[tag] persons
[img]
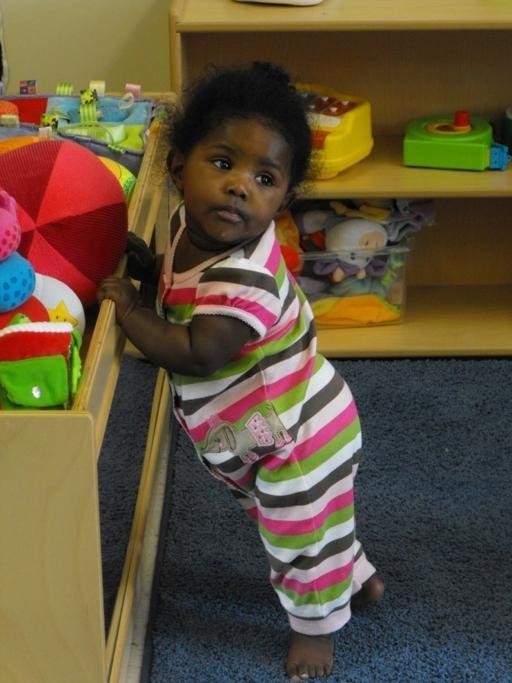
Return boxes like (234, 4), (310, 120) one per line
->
(93, 59), (387, 683)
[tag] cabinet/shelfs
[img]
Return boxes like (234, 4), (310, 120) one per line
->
(171, 1), (510, 356)
(1, 88), (175, 683)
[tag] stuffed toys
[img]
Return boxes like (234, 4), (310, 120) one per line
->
(295, 198), (386, 282)
